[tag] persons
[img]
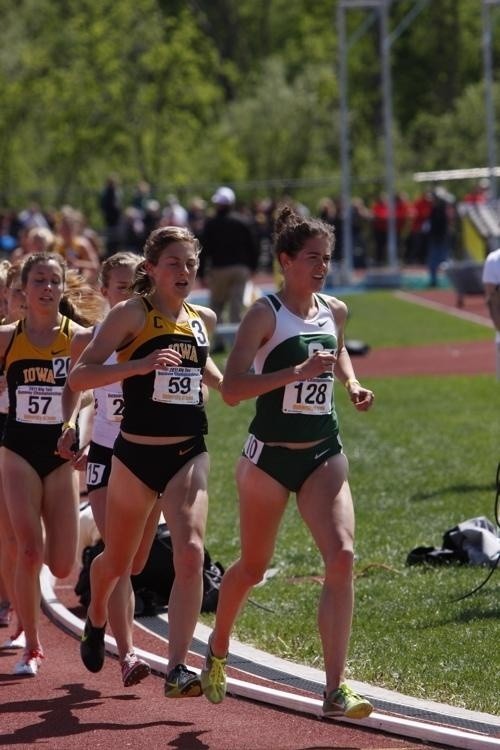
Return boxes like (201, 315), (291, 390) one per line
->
(68, 226), (223, 699)
(0, 258), (39, 649)
(57, 249), (152, 686)
(0, 254), (87, 679)
(200, 204), (374, 723)
(0, 578), (13, 628)
(482, 245), (499, 376)
(2, 173), (499, 327)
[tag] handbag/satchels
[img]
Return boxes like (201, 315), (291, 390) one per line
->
(405, 516), (500, 568)
(75, 522), (224, 618)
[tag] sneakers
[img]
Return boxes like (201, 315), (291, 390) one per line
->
(0, 623), (26, 648)
(80, 617), (107, 673)
(164, 667), (203, 698)
(121, 653), (151, 687)
(200, 633), (230, 704)
(13, 646), (43, 675)
(321, 684), (374, 719)
(0, 599), (14, 626)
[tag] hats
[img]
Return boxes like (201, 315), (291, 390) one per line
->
(210, 185), (236, 206)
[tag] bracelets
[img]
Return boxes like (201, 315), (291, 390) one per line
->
(344, 379), (360, 388)
(60, 423), (74, 433)
(216, 380), (221, 390)
